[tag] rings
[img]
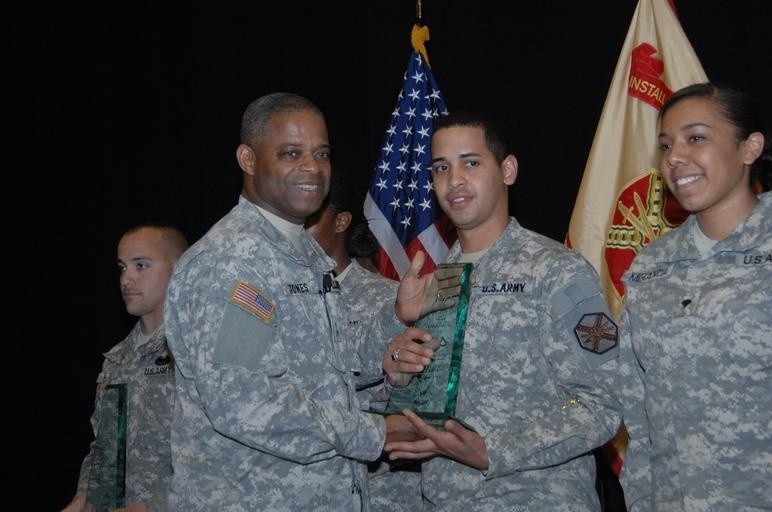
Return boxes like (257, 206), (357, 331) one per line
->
(390, 348), (401, 364)
(437, 289), (442, 302)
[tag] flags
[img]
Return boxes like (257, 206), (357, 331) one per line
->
(565, 2), (762, 478)
(360, 20), (460, 283)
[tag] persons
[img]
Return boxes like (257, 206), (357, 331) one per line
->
(618, 79), (772, 510)
(166, 89), (460, 511)
(370, 113), (621, 511)
(60, 223), (190, 512)
(305, 150), (405, 394)
